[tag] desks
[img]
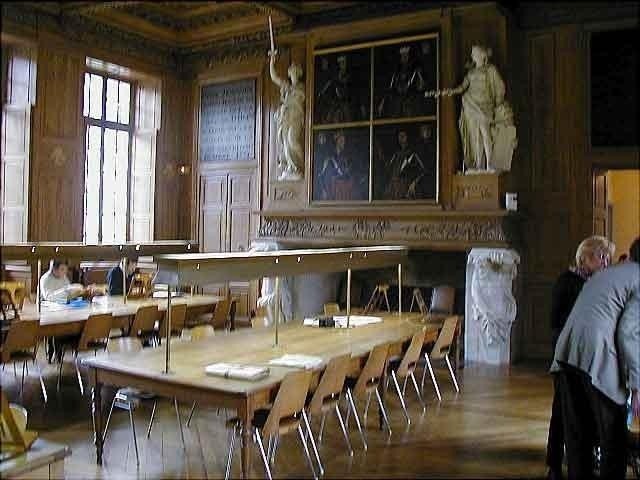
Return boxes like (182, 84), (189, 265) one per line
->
(1, 241), (197, 311)
(153, 244), (409, 372)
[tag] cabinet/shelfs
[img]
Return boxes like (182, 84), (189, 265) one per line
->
(592, 166), (640, 267)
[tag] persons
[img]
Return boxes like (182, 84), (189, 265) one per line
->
(544, 237), (616, 479)
(381, 127), (424, 199)
(319, 54), (368, 120)
(436, 44), (513, 172)
(37, 258), (94, 302)
(105, 254), (139, 295)
(548, 237), (640, 480)
(267, 49), (304, 180)
(382, 45), (426, 117)
(317, 131), (368, 200)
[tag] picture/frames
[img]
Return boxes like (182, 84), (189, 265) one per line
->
(309, 34), (441, 207)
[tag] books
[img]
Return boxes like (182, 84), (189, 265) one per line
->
(204, 362), (272, 379)
